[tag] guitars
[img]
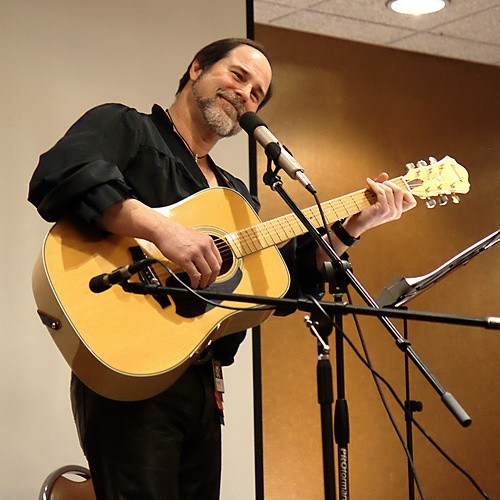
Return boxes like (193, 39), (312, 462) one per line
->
(31, 153), (470, 401)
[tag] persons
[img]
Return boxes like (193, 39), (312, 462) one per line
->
(27, 38), (417, 500)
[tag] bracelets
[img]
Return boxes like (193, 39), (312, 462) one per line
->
(330, 217), (360, 249)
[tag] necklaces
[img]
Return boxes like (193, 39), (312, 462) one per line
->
(165, 108), (208, 160)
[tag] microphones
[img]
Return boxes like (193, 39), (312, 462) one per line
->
(238, 111), (317, 196)
(89, 258), (156, 294)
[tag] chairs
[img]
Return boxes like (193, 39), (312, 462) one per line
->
(40, 466), (96, 500)
